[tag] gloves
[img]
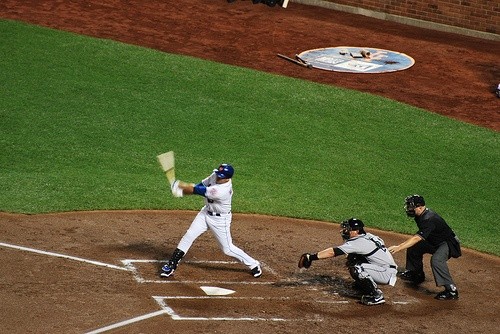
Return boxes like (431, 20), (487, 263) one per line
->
(172, 180), (183, 199)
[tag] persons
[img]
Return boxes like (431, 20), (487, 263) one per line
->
(157, 151), (262, 279)
(387, 194), (463, 301)
(297, 218), (398, 306)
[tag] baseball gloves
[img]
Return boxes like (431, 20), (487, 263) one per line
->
(298, 253), (312, 270)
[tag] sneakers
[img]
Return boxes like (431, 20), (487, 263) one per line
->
(367, 295), (385, 305)
(397, 269), (426, 280)
(352, 281), (372, 291)
(251, 266), (262, 277)
(160, 262), (174, 277)
(435, 289), (458, 300)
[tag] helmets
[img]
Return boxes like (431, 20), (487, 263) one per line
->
(404, 194), (425, 208)
(340, 218), (364, 230)
(213, 163), (235, 179)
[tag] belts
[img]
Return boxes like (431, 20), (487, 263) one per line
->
(208, 211), (220, 217)
(390, 265), (396, 269)
